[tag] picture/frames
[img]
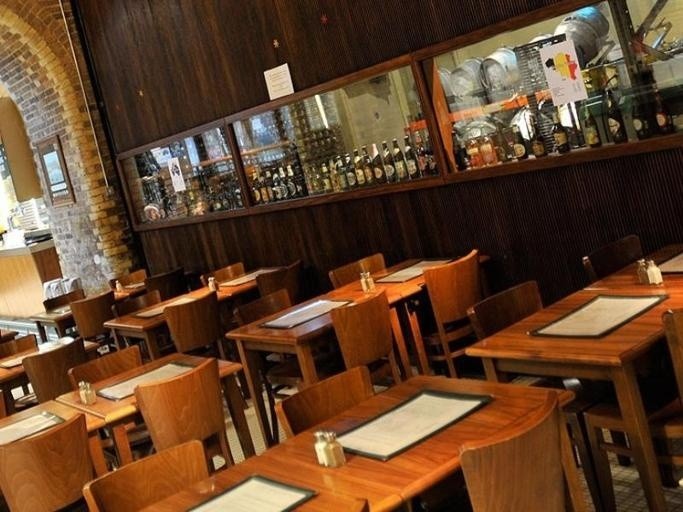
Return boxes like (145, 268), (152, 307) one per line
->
(31, 132), (78, 211)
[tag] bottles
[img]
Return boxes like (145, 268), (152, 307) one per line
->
(631, 95), (650, 140)
(392, 137), (409, 181)
(271, 166), (282, 200)
(278, 167), (291, 200)
(450, 131), (468, 172)
(414, 130), (429, 176)
(264, 168), (276, 201)
(329, 158), (341, 193)
(464, 127), (482, 166)
(335, 155), (350, 192)
(360, 145), (376, 186)
(529, 115), (547, 159)
(79, 382), (88, 405)
(320, 162), (334, 194)
(651, 77), (674, 134)
(309, 163), (324, 195)
(287, 164), (299, 199)
(478, 124), (500, 167)
(363, 271), (376, 290)
(324, 431), (346, 469)
(257, 169), (271, 204)
(636, 258), (651, 286)
(252, 169), (266, 205)
(211, 276), (219, 291)
(403, 134), (419, 179)
(512, 124), (527, 161)
(551, 111), (571, 154)
(208, 278), (215, 291)
(312, 431), (329, 466)
(303, 162), (315, 194)
(293, 164), (307, 198)
(605, 85), (628, 143)
(115, 279), (122, 292)
(352, 149), (367, 188)
(495, 122), (511, 162)
(424, 128), (441, 175)
(359, 272), (368, 292)
(581, 99), (600, 148)
(371, 142), (387, 186)
(381, 140), (397, 184)
(345, 152), (359, 190)
(83, 382), (96, 404)
(646, 258), (664, 285)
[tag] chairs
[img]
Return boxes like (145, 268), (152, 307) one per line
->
(66, 343), (153, 465)
(0, 333), (39, 401)
(83, 438), (211, 512)
(231, 286), (325, 442)
(465, 279), (604, 512)
(163, 292), (251, 411)
(274, 364), (376, 438)
(108, 267), (150, 319)
(68, 289), (115, 357)
(421, 246), (483, 379)
(255, 255), (310, 307)
(111, 287), (169, 353)
(14, 334), (90, 413)
(142, 264), (189, 302)
(42, 287), (87, 340)
(457, 388), (569, 512)
(327, 252), (387, 290)
(133, 355), (236, 477)
(581, 306), (682, 512)
(581, 233), (644, 284)
(0, 411), (95, 512)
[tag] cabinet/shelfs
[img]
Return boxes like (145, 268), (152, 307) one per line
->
(223, 51), (446, 216)
(114, 1), (250, 233)
(411, 1), (683, 185)
